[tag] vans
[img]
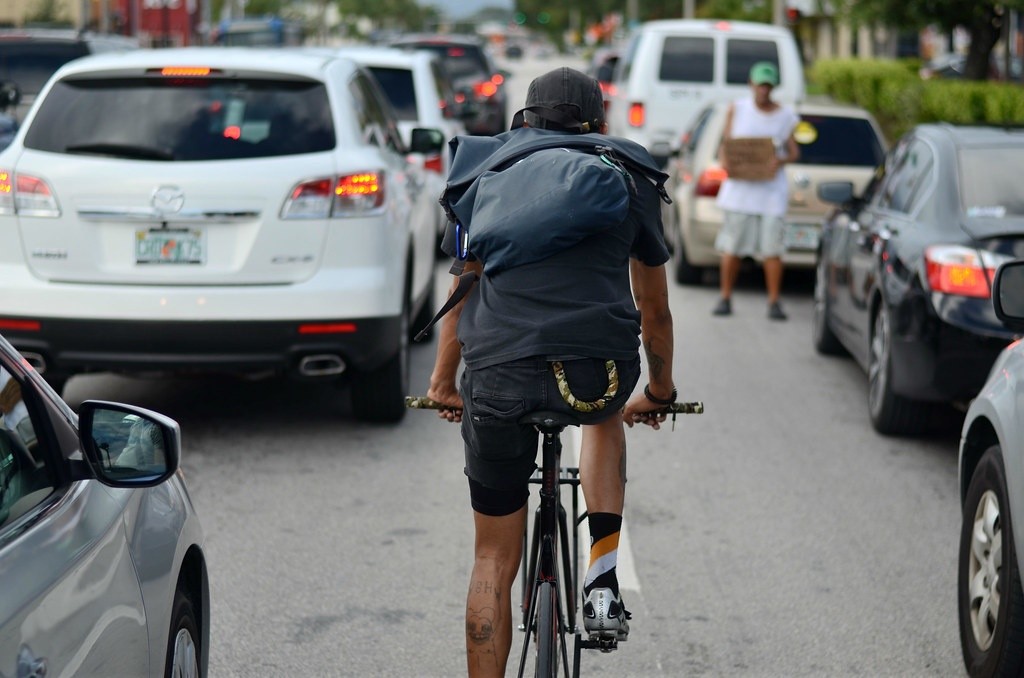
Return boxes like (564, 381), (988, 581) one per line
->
(602, 19), (808, 168)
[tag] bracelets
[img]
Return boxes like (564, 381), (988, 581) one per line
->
(644, 383), (676, 406)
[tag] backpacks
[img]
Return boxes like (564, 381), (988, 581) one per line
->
(415, 129), (671, 344)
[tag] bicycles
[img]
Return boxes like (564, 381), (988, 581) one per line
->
(403, 393), (705, 677)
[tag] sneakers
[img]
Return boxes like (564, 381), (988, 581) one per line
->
(581, 584), (628, 642)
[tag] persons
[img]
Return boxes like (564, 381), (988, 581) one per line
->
(425, 65), (677, 678)
(712, 62), (803, 319)
(112, 416), (164, 470)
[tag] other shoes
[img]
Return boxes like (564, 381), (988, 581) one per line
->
(770, 304), (786, 320)
(713, 298), (731, 313)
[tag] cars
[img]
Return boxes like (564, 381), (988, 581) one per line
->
(811, 122), (1023, 439)
(957, 260), (1023, 678)
(0, 29), (143, 150)
(1, 335), (210, 678)
(1, 47), (449, 424)
(389, 35), (506, 134)
(332, 44), (470, 212)
(650, 101), (890, 289)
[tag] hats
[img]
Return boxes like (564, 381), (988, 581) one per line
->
(524, 67), (606, 131)
(749, 61), (780, 87)
